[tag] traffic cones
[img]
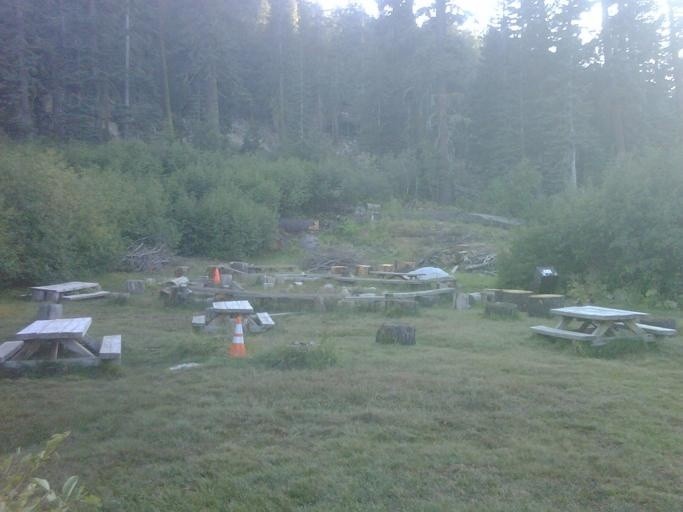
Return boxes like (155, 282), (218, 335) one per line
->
(227, 314), (249, 359)
(211, 267), (222, 284)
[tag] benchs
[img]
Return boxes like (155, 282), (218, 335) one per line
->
(371, 271), (426, 280)
(0, 281), (121, 368)
(530, 306), (677, 348)
(192, 299), (276, 331)
